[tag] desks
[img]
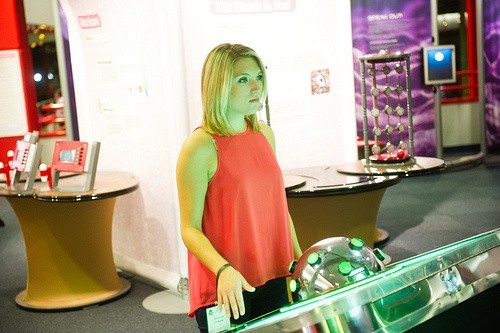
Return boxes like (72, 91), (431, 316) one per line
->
(0, 171), (139, 309)
(282, 157), (445, 254)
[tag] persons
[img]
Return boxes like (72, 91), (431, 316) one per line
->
(176, 41), (302, 333)
